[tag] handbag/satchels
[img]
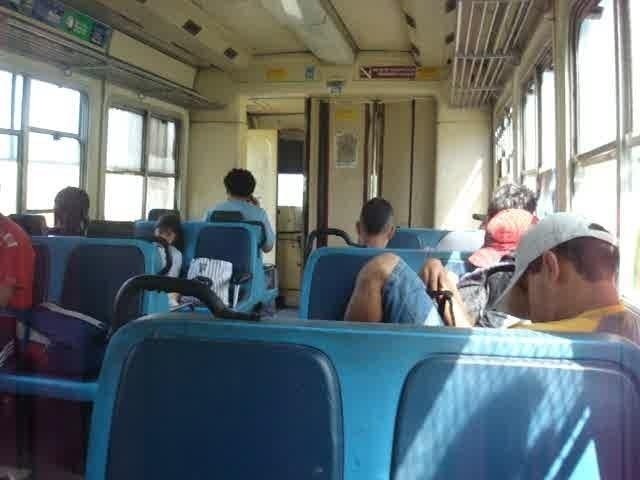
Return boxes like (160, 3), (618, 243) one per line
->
(15, 302), (108, 378)
(181, 257), (233, 308)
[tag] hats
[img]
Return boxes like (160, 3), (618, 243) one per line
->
(468, 208), (541, 268)
(494, 212), (618, 320)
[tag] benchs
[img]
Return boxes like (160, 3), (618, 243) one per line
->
(2, 229), (160, 480)
(131, 208), (280, 303)
(375, 225), (454, 247)
(74, 310), (640, 480)
(298, 245), (487, 330)
(6, 209), (195, 315)
(116, 220), (257, 318)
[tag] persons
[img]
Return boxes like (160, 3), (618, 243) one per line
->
(0, 212), (36, 366)
(202, 168), (275, 253)
(53, 185), (90, 237)
(152, 213), (183, 308)
(492, 211), (640, 347)
(434, 183), (537, 251)
(355, 196), (395, 249)
(342, 208), (541, 329)
